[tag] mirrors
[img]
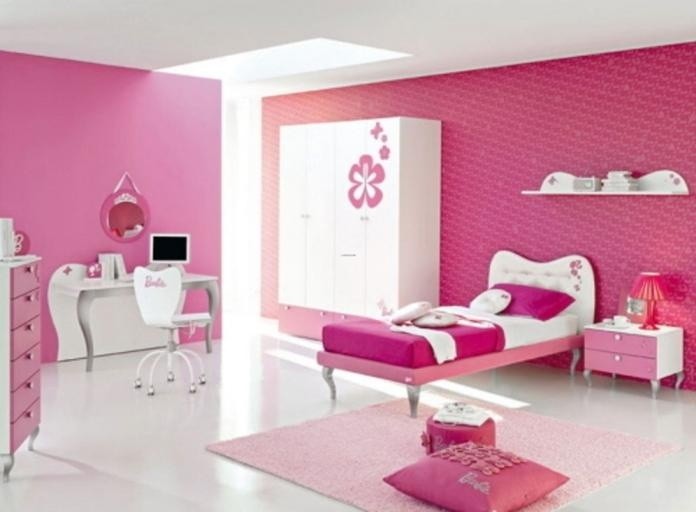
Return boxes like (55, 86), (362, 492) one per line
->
(100, 189), (152, 245)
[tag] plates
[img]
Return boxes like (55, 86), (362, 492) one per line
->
(603, 322), (630, 329)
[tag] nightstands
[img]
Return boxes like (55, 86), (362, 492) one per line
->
(582, 320), (686, 399)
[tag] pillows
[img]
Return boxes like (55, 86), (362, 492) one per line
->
(382, 441), (570, 512)
(393, 300), (430, 321)
(489, 283), (577, 323)
(470, 287), (513, 315)
(416, 310), (457, 328)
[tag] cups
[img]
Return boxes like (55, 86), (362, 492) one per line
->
(1, 218), (16, 259)
(614, 315), (632, 326)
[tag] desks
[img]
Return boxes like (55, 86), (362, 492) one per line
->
(52, 272), (220, 374)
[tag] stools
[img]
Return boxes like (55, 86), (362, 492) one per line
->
(421, 408), (497, 456)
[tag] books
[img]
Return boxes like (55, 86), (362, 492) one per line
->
(600, 171), (637, 192)
(99, 253), (127, 282)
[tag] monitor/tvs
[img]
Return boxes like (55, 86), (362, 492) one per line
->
(149, 231), (192, 268)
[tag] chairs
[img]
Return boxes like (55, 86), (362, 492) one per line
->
(132, 264), (212, 396)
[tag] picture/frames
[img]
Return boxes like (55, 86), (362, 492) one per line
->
(617, 288), (649, 323)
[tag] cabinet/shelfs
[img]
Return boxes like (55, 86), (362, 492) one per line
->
(278, 122), (335, 314)
(335, 117), (443, 321)
(0, 253), (43, 483)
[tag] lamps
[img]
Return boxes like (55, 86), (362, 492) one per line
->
(628, 271), (666, 330)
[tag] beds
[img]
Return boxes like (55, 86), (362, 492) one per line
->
(316, 248), (597, 417)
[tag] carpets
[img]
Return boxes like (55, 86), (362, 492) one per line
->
(205, 386), (680, 512)
